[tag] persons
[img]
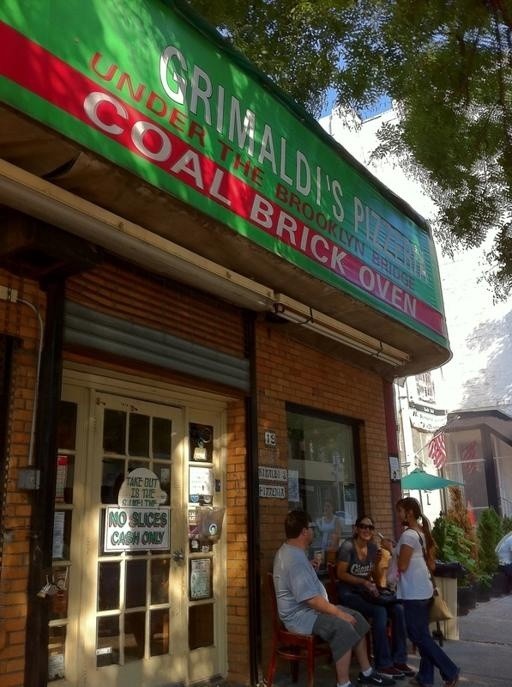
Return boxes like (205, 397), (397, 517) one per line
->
(379, 497), (461, 685)
(270, 509), (397, 686)
(314, 498), (346, 550)
(334, 512), (415, 679)
(495, 530), (512, 595)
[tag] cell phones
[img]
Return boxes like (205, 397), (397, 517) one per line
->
(377, 531), (383, 540)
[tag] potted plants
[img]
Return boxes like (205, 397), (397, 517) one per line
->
(432, 505), (512, 617)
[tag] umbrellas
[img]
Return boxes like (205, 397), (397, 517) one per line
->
(400, 465), (466, 513)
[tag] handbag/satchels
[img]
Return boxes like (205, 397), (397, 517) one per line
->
(429, 594), (453, 622)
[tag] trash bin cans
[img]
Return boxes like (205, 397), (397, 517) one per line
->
(431, 563), (459, 641)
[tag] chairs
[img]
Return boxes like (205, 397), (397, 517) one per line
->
(266, 565), (394, 687)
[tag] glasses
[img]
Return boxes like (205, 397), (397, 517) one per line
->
(307, 526), (316, 530)
(357, 524), (374, 530)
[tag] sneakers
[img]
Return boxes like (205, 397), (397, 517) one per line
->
(336, 680), (353, 687)
(443, 670), (462, 687)
(380, 662), (415, 678)
(408, 676), (433, 687)
(356, 669), (396, 687)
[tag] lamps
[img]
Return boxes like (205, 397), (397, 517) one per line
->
(0, 158), (274, 373)
(274, 293), (411, 369)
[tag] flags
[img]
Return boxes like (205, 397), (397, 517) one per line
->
(427, 433), (447, 468)
(461, 441), (481, 474)
